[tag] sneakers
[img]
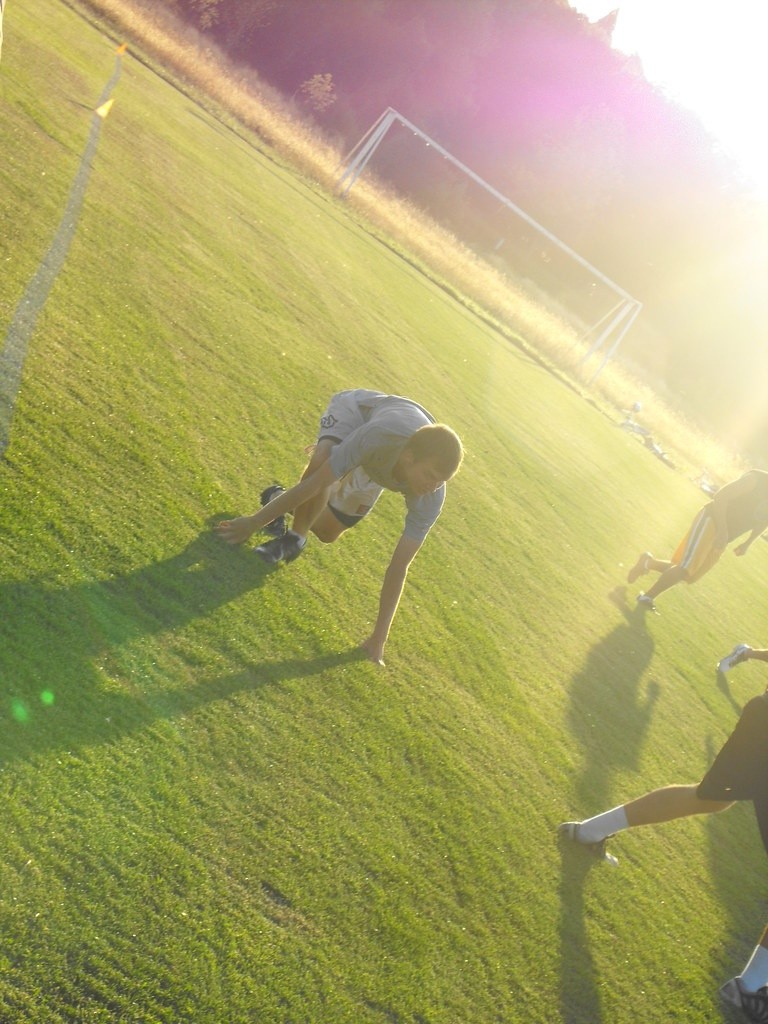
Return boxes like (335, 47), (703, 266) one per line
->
(560, 822), (618, 867)
(718, 977), (768, 1024)
(629, 552), (653, 583)
(717, 644), (750, 672)
(637, 592), (661, 616)
(255, 531), (307, 565)
(260, 485), (286, 536)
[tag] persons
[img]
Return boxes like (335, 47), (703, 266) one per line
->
(559, 690), (768, 1024)
(631, 470), (768, 611)
(214, 389), (463, 666)
(717, 644), (768, 675)
